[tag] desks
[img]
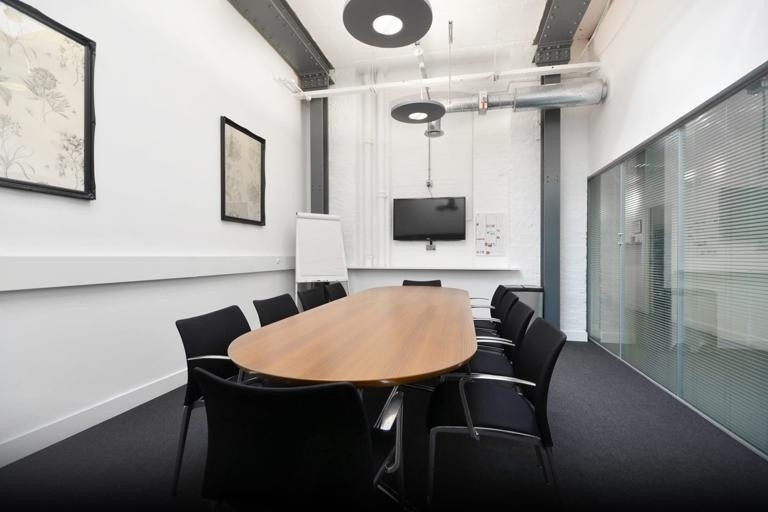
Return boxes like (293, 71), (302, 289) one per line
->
(225, 286), (479, 395)
(502, 284), (545, 319)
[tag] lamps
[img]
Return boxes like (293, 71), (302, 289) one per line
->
(341, 0), (447, 125)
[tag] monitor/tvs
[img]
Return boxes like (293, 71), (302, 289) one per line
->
(393, 196), (465, 240)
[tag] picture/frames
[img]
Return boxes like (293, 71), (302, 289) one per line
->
(220, 115), (267, 226)
(0, 0), (97, 200)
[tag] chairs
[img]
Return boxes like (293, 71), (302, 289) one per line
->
(426, 317), (570, 504)
(252, 293), (299, 328)
(475, 292), (518, 353)
(170, 306), (300, 493)
(402, 280), (441, 286)
(469, 286), (506, 329)
(438, 301), (535, 383)
(326, 282), (348, 303)
(197, 366), (406, 512)
(298, 286), (327, 311)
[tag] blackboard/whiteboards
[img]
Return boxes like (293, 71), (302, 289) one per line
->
(295, 214), (348, 284)
